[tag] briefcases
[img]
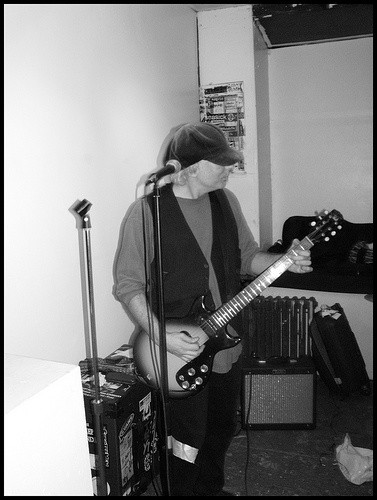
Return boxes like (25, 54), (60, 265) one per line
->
(240, 356), (316, 429)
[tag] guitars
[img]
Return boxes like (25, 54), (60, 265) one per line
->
(130, 209), (344, 396)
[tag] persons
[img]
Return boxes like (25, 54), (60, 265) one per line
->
(111, 119), (314, 500)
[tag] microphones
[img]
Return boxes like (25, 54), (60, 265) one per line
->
(145, 159), (181, 186)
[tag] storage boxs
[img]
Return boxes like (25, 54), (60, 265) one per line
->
(81, 380), (158, 496)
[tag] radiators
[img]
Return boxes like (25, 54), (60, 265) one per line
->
(243, 295), (318, 361)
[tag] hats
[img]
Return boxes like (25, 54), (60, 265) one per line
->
(169, 123), (243, 170)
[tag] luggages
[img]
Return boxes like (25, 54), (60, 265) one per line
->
(311, 303), (370, 398)
